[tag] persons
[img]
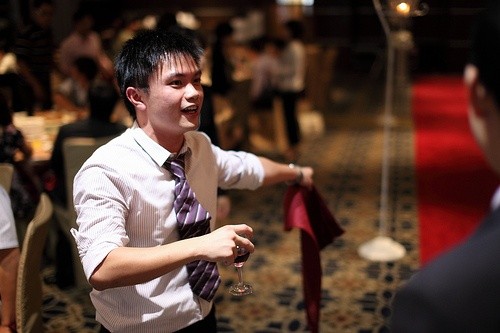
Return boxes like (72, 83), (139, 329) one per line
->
(0, 0), (307, 169)
(0, 184), (22, 333)
(388, 54), (500, 333)
(70, 33), (316, 333)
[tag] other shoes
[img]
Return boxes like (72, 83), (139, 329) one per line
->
(44, 274), (59, 286)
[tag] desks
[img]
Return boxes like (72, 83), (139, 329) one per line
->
(15, 111), (90, 167)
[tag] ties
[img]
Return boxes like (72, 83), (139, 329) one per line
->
(162, 154), (220, 302)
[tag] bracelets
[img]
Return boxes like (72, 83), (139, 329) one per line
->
(284, 163), (304, 188)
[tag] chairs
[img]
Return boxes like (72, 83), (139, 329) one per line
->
(51, 137), (111, 286)
(14, 193), (52, 333)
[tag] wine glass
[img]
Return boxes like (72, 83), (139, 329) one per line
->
(229, 233), (254, 296)
